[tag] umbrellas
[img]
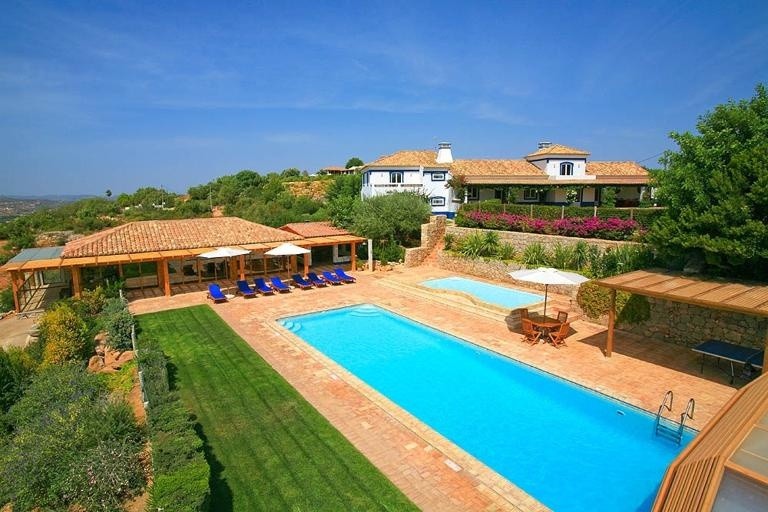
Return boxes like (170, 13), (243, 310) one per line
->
(508, 266), (591, 318)
(196, 248), (252, 294)
(264, 243), (311, 286)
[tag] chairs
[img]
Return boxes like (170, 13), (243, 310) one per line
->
(519, 308), (570, 350)
(208, 269), (356, 304)
